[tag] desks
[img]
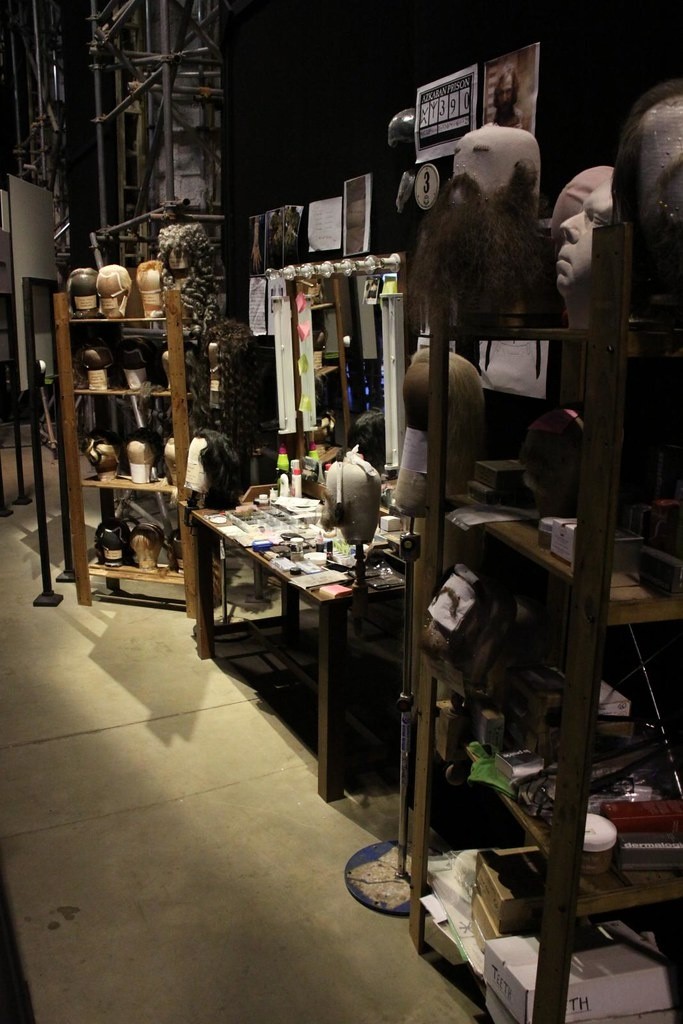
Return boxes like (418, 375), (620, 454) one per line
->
(54, 291), (202, 620)
(191, 506), (409, 802)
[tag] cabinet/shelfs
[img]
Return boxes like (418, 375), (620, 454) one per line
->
(411, 224), (683, 1024)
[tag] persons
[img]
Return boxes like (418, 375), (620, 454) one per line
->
(556, 183), (613, 329)
(610, 79), (683, 301)
(70, 335), (181, 391)
(326, 461), (382, 545)
(63, 260), (168, 319)
(493, 67), (523, 130)
(404, 349), (485, 492)
(417, 562), (517, 702)
(80, 426), (232, 493)
(519, 409), (585, 497)
(95, 517), (185, 577)
(415, 121), (541, 317)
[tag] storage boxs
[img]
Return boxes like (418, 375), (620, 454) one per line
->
(482, 923), (674, 1024)
(472, 886), (503, 939)
(475, 845), (549, 935)
